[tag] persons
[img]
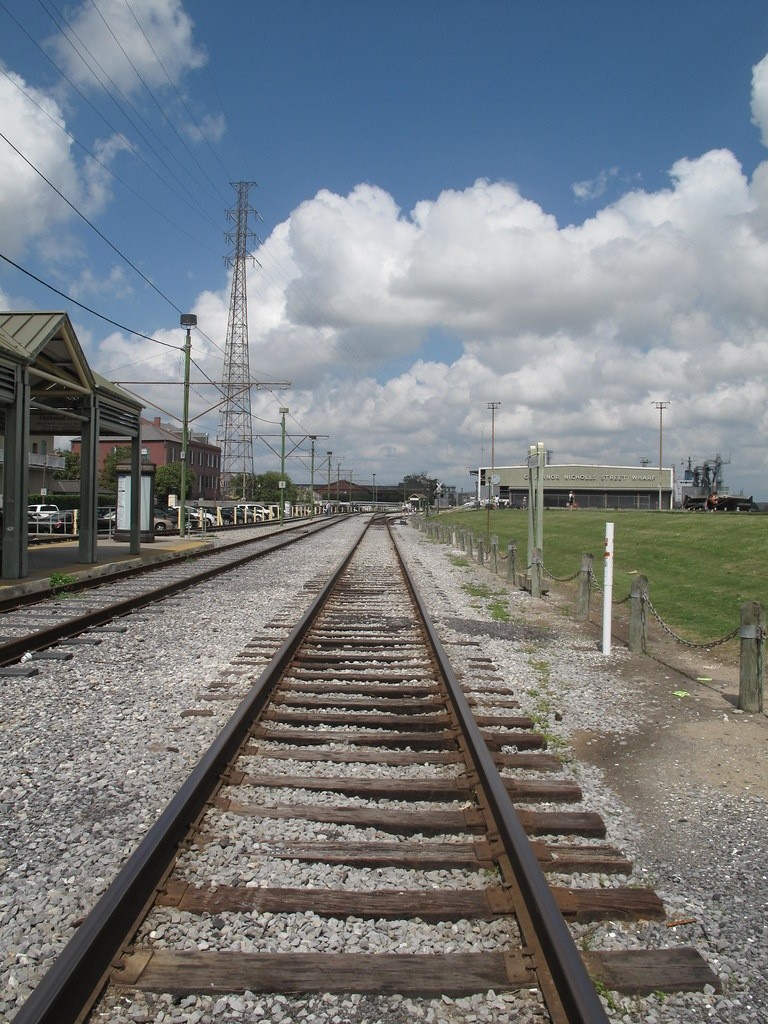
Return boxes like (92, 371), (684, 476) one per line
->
(523, 497), (527, 508)
(480, 495), (499, 507)
(326, 501), (331, 517)
(568, 491), (575, 511)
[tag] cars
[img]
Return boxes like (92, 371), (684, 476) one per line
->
(27, 504), (283, 533)
